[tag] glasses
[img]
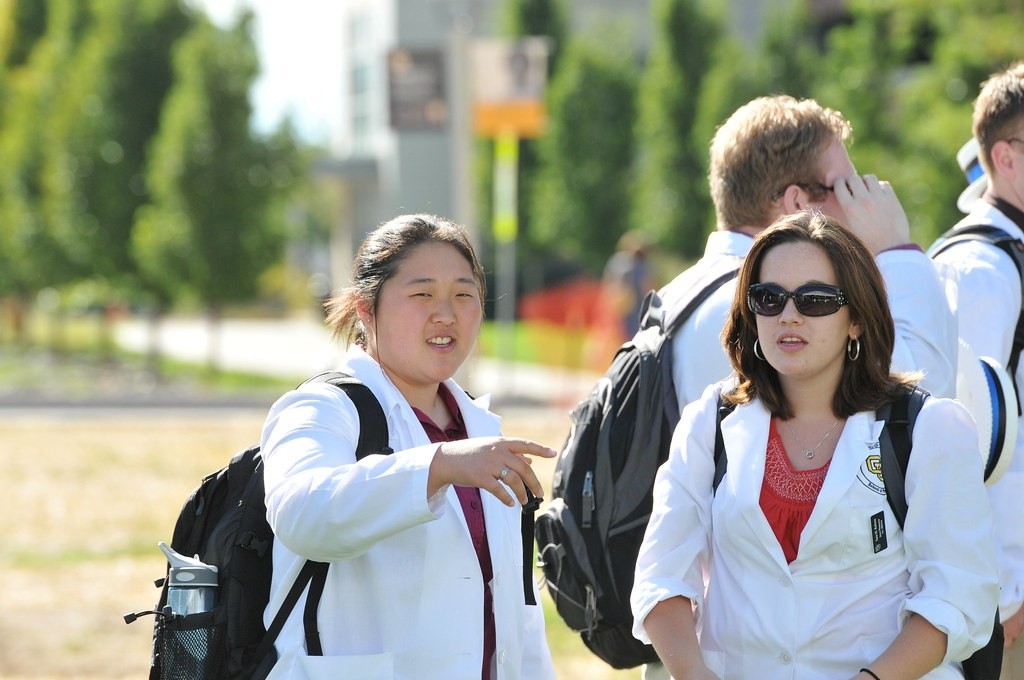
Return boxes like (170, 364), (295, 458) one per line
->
(745, 283), (851, 317)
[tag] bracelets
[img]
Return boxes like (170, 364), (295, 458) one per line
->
(859, 668), (880, 680)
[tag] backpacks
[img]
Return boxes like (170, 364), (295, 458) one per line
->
(534, 268), (742, 669)
(123, 369), (393, 680)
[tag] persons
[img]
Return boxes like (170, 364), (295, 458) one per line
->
(261, 213), (557, 679)
(629, 211), (1000, 680)
(641, 95), (960, 680)
(927, 61), (1023, 680)
(601, 229), (662, 349)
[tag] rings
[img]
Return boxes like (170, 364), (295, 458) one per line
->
(498, 466), (510, 480)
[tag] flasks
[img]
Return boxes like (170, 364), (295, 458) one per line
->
(156, 541), (218, 680)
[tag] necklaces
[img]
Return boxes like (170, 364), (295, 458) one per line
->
(781, 410), (839, 459)
